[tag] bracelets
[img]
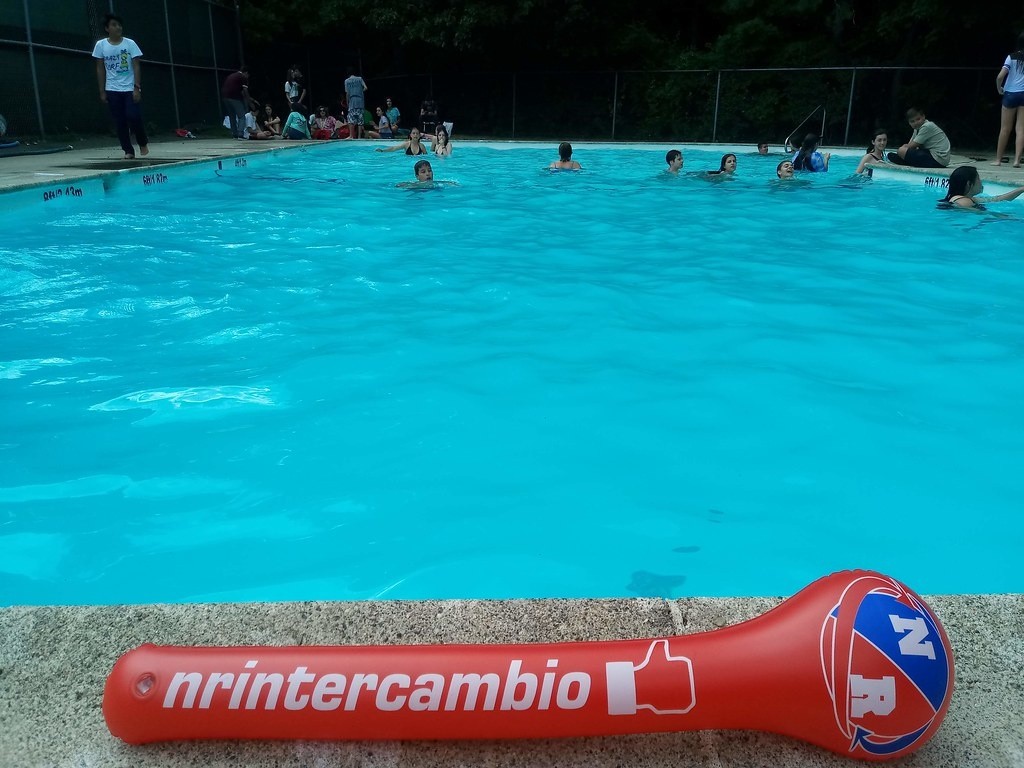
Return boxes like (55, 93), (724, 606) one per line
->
(134, 84), (140, 88)
(442, 146), (445, 149)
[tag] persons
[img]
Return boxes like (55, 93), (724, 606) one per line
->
(886, 106), (951, 169)
(989, 32), (1024, 168)
(430, 125), (453, 155)
(414, 160), (433, 182)
(666, 149), (684, 175)
(549, 142), (581, 169)
(220, 63), (402, 140)
(91, 13), (150, 160)
(707, 153), (737, 176)
(776, 132), (831, 180)
(375, 126), (428, 156)
(856, 128), (888, 174)
(757, 142), (769, 155)
(935, 165), (1024, 210)
